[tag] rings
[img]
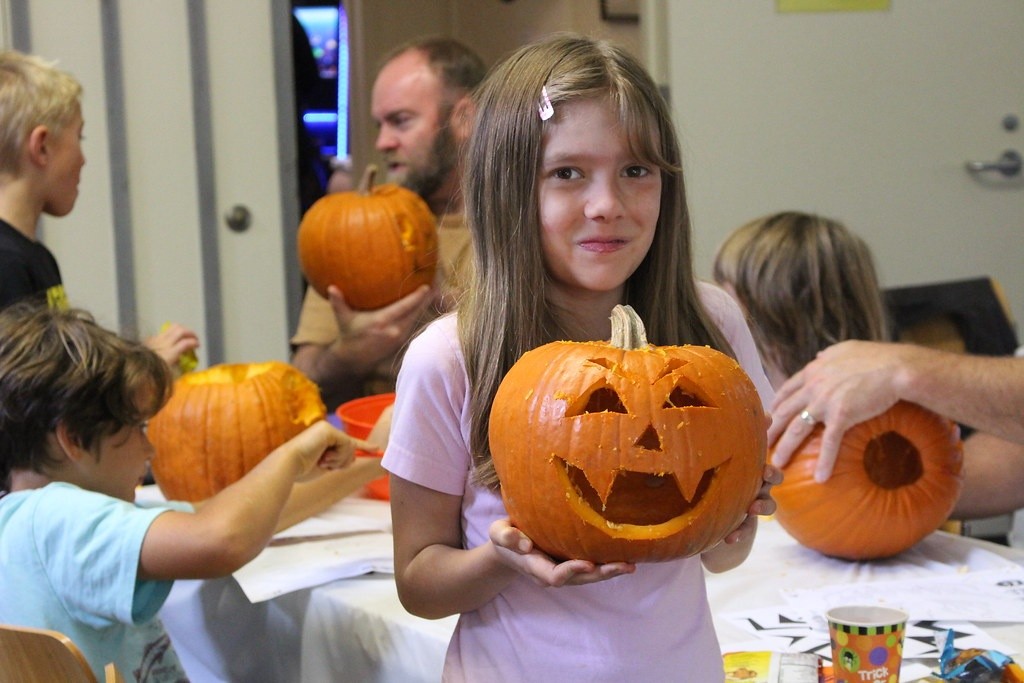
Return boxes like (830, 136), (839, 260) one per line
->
(801, 408), (819, 426)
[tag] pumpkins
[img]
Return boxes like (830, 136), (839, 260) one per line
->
(771, 398), (965, 566)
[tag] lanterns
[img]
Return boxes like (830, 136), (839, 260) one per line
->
(144, 341), (329, 512)
(765, 387), (966, 565)
(485, 303), (769, 567)
(296, 165), (441, 315)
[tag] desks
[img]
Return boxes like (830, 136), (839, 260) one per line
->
(127, 453), (1024, 682)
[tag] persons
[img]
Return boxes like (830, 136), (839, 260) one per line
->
(763, 291), (1024, 556)
(711, 209), (888, 398)
(0, 310), (405, 683)
(2, 39), (201, 504)
(288, 34), (502, 418)
(379, 36), (780, 683)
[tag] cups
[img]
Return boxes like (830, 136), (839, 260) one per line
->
(826, 606), (908, 683)
